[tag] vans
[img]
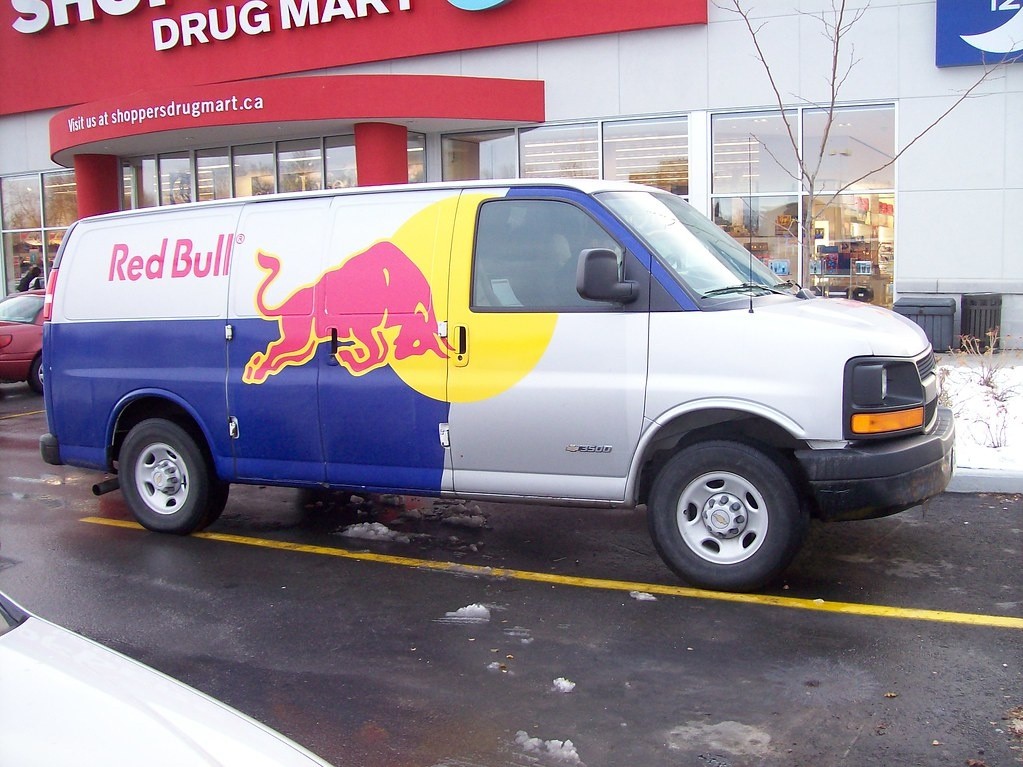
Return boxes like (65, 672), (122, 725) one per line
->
(39, 172), (955, 591)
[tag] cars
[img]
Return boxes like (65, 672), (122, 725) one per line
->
(0, 289), (51, 395)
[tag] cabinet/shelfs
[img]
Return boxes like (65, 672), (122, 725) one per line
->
(813, 239), (894, 306)
(732, 234), (810, 290)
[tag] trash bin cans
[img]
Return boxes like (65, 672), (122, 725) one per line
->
(962, 292), (1004, 353)
(892, 298), (955, 351)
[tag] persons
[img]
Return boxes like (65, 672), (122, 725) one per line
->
(20, 257), (32, 273)
(16, 267), (41, 293)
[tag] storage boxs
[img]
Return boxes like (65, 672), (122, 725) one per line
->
(811, 286), (849, 298)
(810, 249), (874, 275)
(768, 258), (791, 277)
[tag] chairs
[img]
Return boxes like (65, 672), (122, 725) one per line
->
(535, 234), (575, 265)
